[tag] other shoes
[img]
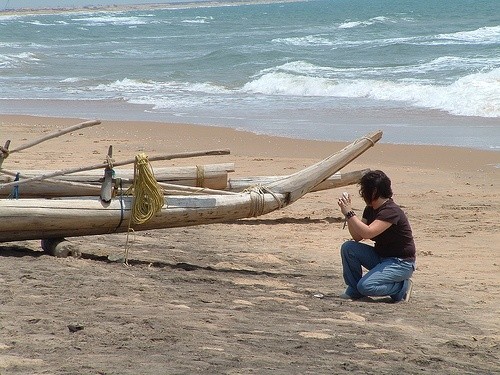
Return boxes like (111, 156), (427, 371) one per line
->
(338, 293), (351, 299)
(403, 279), (414, 302)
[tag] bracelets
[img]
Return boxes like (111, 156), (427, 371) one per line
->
(346, 211), (356, 219)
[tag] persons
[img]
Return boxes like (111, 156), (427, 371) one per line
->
(337, 170), (416, 303)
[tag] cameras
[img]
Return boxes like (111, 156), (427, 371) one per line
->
(343, 192), (349, 199)
